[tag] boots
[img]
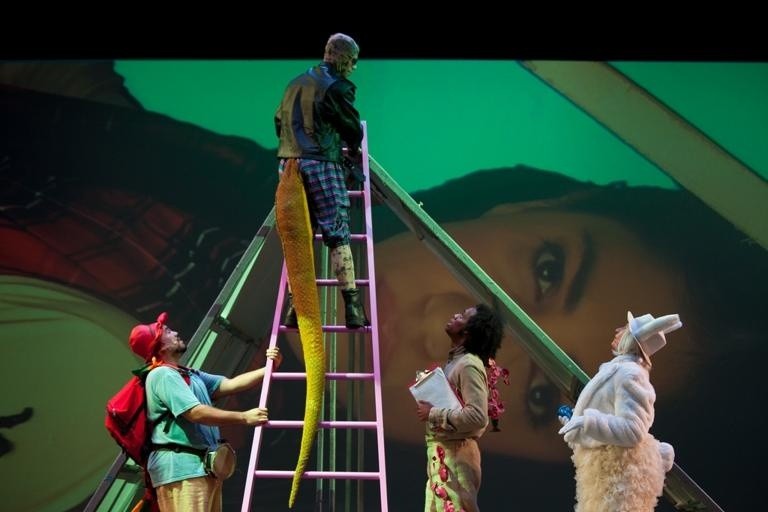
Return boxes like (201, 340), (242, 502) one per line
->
(340, 288), (369, 327)
(283, 294), (300, 328)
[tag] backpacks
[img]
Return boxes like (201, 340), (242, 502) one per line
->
(103, 365), (198, 467)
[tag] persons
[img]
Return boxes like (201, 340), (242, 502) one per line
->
(127, 312), (283, 512)
(0, 83), (768, 511)
(557, 309), (683, 512)
(274, 34), (371, 328)
(417, 304), (505, 512)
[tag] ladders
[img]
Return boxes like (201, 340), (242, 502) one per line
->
(84, 138), (731, 512)
(240, 122), (389, 511)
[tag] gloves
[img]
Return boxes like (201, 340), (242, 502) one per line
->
(558, 415), (583, 435)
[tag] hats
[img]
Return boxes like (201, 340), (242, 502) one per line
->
(129, 311), (168, 364)
(625, 309), (684, 369)
